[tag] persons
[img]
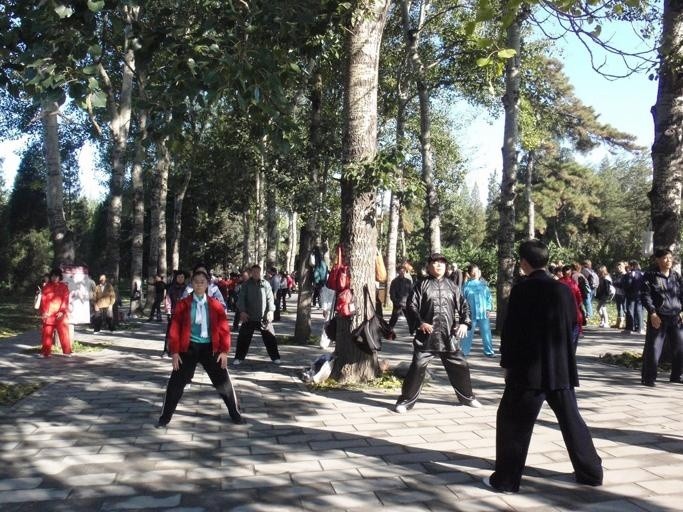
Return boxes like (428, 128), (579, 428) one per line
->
(33, 263), (117, 360)
(480, 239), (604, 493)
(154, 270), (249, 427)
(638, 247), (682, 388)
(143, 243), (648, 382)
(392, 253), (484, 413)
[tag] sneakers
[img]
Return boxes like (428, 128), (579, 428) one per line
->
(38, 354), (52, 359)
(469, 400), (482, 408)
(643, 381), (655, 387)
(64, 353), (73, 357)
(488, 355), (499, 359)
(233, 419), (246, 425)
(110, 331), (118, 334)
(156, 319), (161, 321)
(584, 320), (645, 335)
(156, 425), (165, 430)
(483, 477), (513, 494)
(395, 405), (409, 414)
(670, 376), (682, 383)
(274, 360), (280, 364)
(93, 331), (100, 335)
(233, 359), (242, 365)
(147, 318), (153, 320)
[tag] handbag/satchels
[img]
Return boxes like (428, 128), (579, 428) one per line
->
(324, 247), (395, 356)
